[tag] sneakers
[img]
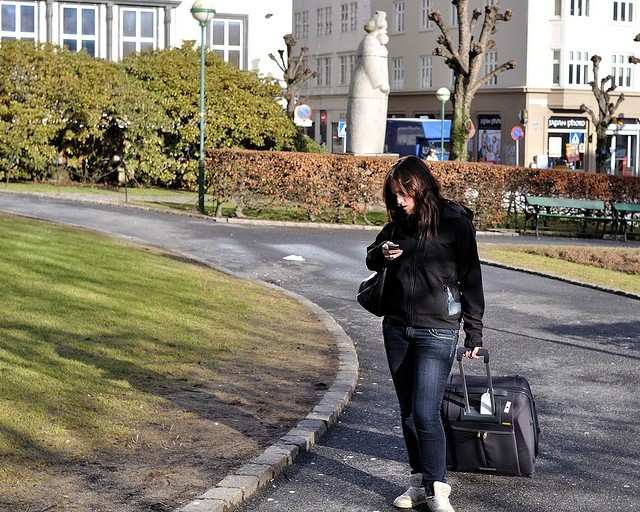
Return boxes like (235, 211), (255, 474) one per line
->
(393, 472), (426, 508)
(425, 480), (454, 511)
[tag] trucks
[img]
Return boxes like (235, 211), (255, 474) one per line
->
(386, 118), (451, 160)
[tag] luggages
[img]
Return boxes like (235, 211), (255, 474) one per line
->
(443, 348), (540, 479)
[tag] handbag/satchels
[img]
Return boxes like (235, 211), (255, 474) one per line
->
(357, 263), (390, 317)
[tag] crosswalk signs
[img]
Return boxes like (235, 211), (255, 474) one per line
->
(338, 122), (347, 137)
(570, 131), (582, 144)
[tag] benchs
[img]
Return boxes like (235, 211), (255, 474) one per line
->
(608, 200), (639, 242)
(520, 192), (613, 241)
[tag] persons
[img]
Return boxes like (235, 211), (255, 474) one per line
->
(346, 10), (391, 154)
(485, 144), (495, 161)
(478, 130), (487, 160)
(491, 136), (500, 155)
(426, 148), (438, 161)
(365, 154), (485, 512)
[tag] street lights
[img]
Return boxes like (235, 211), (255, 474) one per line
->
(436, 87), (451, 161)
(190, 0), (216, 215)
